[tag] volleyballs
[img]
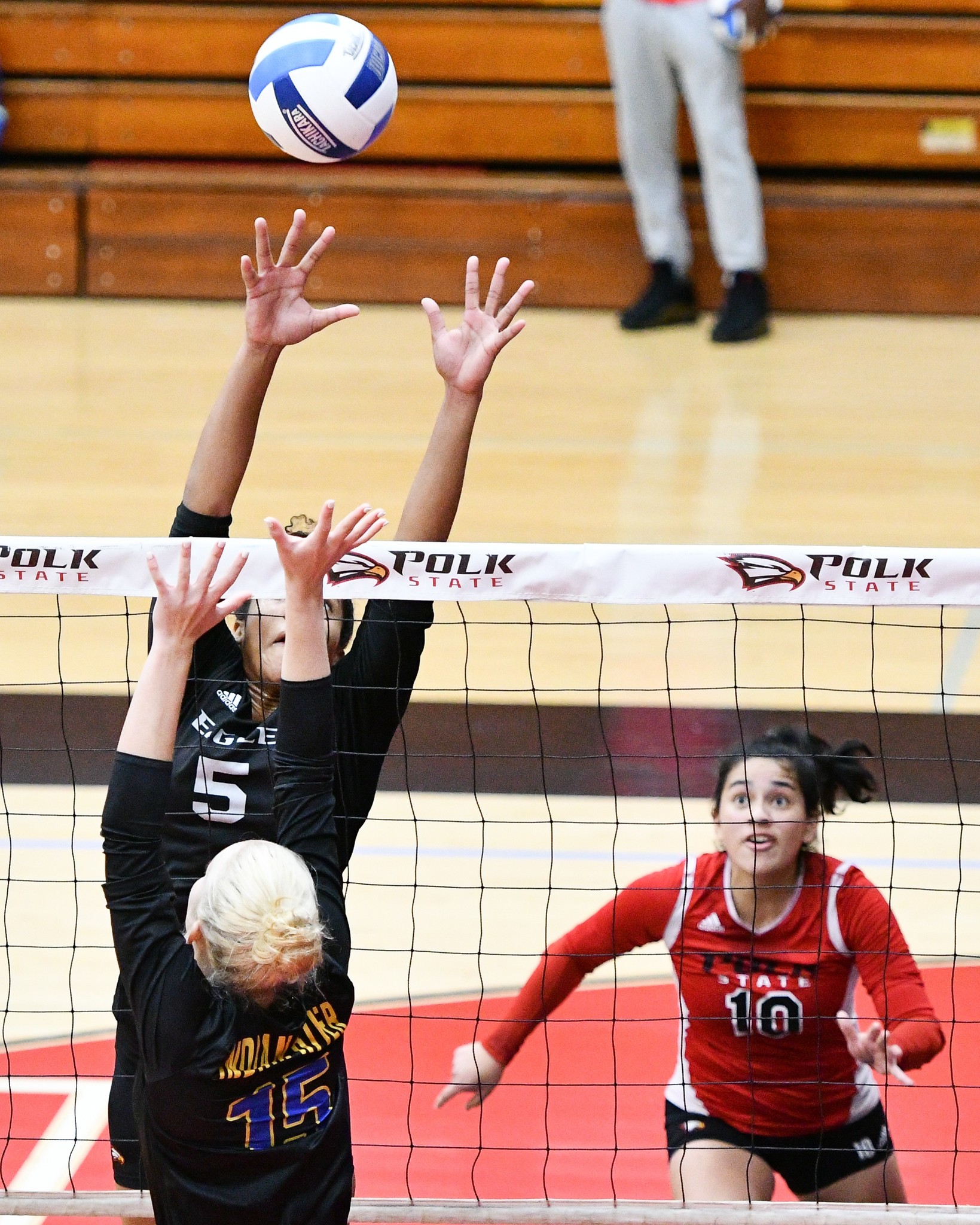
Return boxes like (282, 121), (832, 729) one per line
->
(707, 0), (784, 53)
(249, 13), (398, 163)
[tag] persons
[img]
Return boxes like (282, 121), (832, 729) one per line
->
(108, 501), (385, 1225)
(108, 208), (537, 1225)
(595, 1), (772, 342)
(434, 727), (943, 1205)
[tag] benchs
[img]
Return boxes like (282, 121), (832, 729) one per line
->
(0, 0), (979, 317)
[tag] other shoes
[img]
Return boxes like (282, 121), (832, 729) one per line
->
(622, 258), (700, 328)
(713, 271), (768, 342)
